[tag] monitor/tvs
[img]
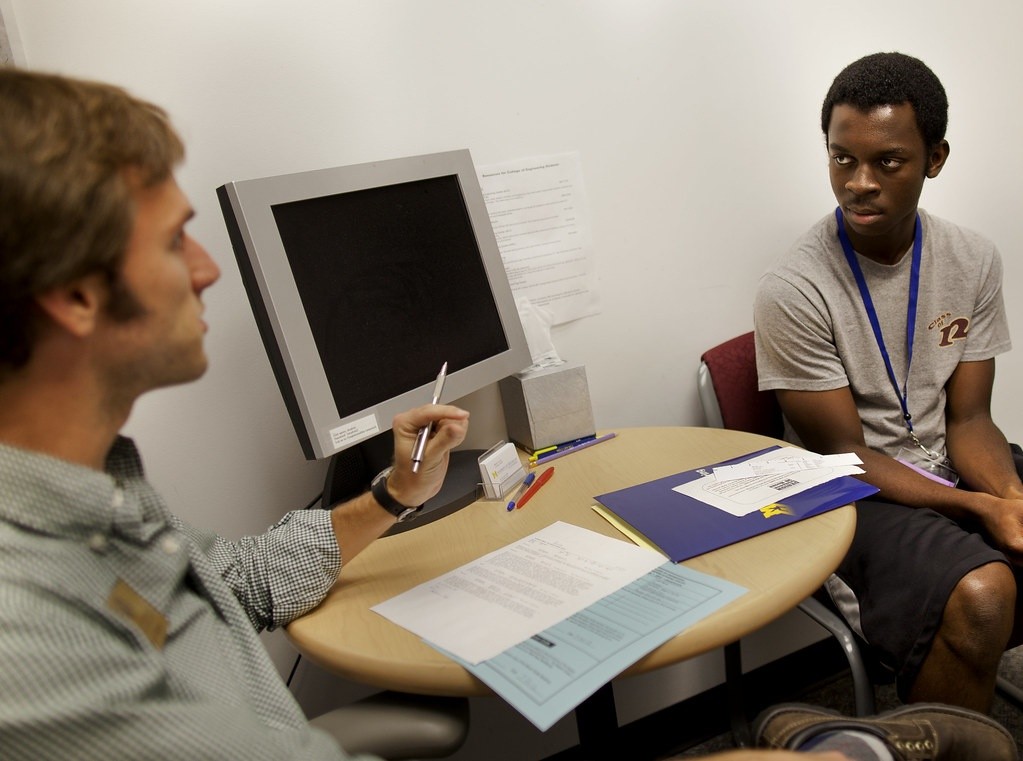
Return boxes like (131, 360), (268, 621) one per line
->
(215, 146), (532, 461)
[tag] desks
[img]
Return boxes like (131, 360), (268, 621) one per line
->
(279, 425), (873, 760)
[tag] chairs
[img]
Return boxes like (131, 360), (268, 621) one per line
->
(697, 330), (1023, 718)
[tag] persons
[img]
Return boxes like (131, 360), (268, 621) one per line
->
(0, 68), (1018, 761)
(753, 51), (1023, 715)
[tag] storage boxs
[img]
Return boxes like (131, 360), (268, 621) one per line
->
(498, 359), (596, 457)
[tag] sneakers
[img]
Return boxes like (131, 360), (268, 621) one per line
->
(751, 702), (1018, 760)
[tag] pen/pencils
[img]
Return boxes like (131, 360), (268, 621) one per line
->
(527, 435), (601, 461)
(507, 470), (536, 512)
(529, 431), (619, 467)
(409, 361), (449, 474)
(515, 467), (555, 508)
